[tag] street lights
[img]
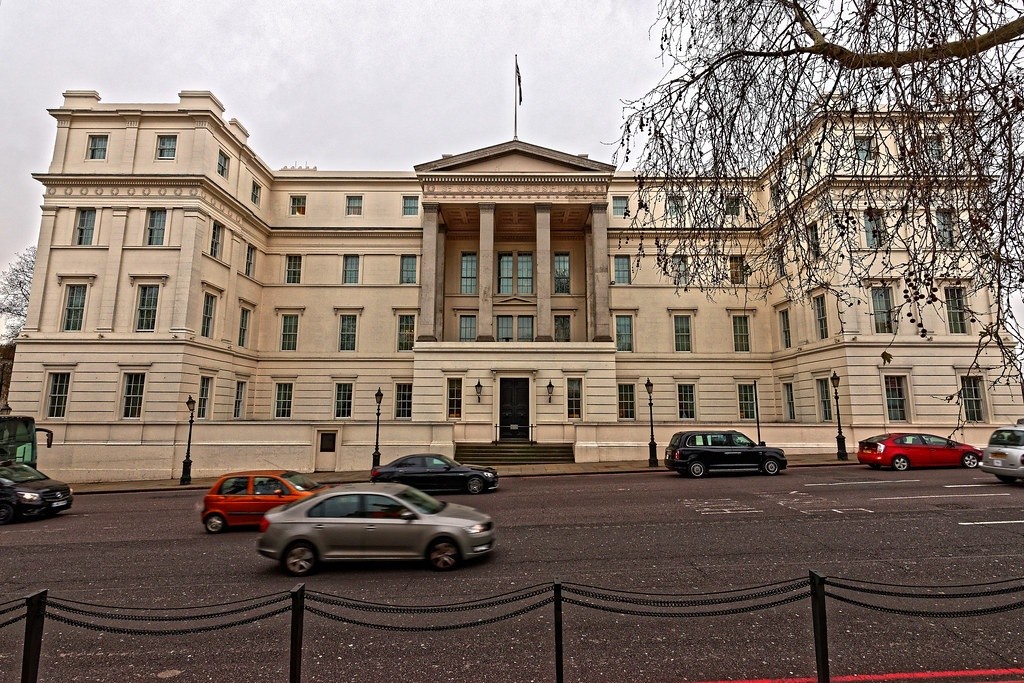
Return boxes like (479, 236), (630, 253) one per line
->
(372, 386), (385, 468)
(830, 370), (849, 461)
(179, 394), (197, 486)
(644, 378), (659, 468)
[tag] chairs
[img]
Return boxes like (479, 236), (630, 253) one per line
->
(997, 434), (1005, 442)
(1007, 434), (1018, 444)
(904, 438), (907, 443)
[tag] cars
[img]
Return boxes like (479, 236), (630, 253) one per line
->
(857, 432), (983, 472)
(0, 463), (75, 527)
(201, 470), (336, 534)
(370, 454), (500, 495)
(256, 482), (497, 578)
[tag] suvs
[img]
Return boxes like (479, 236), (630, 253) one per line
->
(982, 423), (1024, 485)
(664, 430), (788, 480)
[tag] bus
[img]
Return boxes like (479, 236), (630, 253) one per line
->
(0, 413), (54, 470)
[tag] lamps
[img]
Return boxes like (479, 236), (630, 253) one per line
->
(608, 280), (616, 286)
(475, 378), (484, 402)
(546, 379), (554, 403)
